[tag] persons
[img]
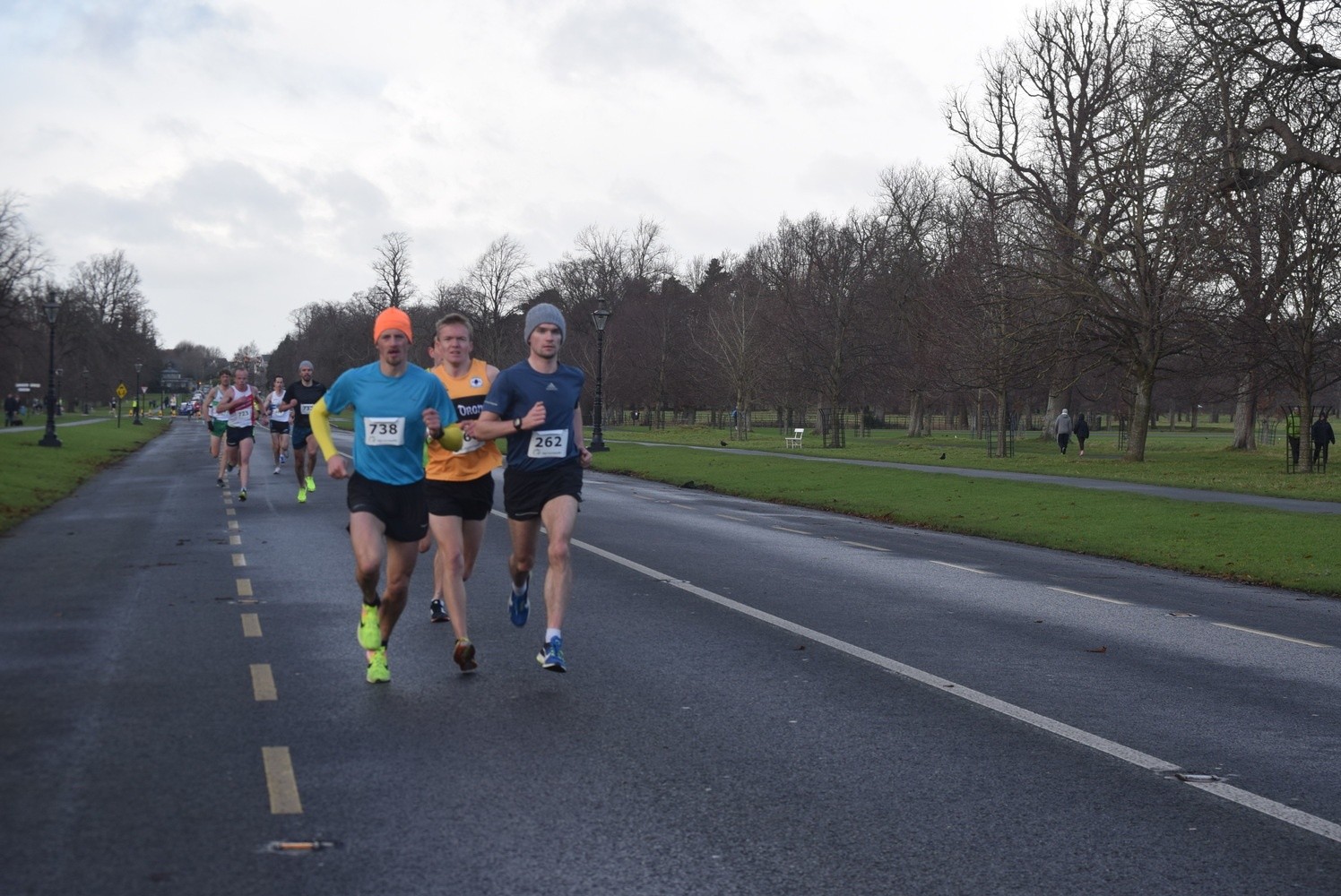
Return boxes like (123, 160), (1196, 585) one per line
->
(732, 408), (743, 430)
(4, 333), (445, 553)
(1055, 408), (1090, 456)
(427, 312), (501, 673)
(474, 303), (593, 672)
(310, 306), (462, 684)
(1287, 406), (1336, 465)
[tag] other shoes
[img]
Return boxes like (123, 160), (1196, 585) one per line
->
(216, 479), (224, 487)
(280, 456), (284, 463)
(239, 490), (247, 501)
(225, 463), (232, 472)
(284, 450), (289, 458)
(275, 467), (280, 474)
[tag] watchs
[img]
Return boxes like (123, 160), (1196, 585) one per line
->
(513, 417), (522, 431)
(432, 424), (444, 440)
(262, 413), (267, 416)
(290, 414), (294, 419)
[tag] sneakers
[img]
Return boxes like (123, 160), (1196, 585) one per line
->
(537, 635), (567, 672)
(357, 592), (382, 650)
(298, 487), (307, 502)
(366, 646), (390, 684)
(430, 599), (449, 622)
(305, 476), (316, 492)
(453, 638), (477, 671)
(509, 571), (532, 627)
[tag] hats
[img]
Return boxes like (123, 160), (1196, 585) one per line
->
(524, 303), (566, 346)
(300, 360), (314, 372)
(374, 307), (412, 345)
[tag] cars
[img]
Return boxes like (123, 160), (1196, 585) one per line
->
(177, 384), (213, 416)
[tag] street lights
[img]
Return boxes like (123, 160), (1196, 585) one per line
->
(585, 295), (612, 452)
(55, 365), (64, 416)
(39, 290), (62, 448)
(133, 358), (144, 425)
(158, 379), (165, 416)
(82, 366), (90, 415)
